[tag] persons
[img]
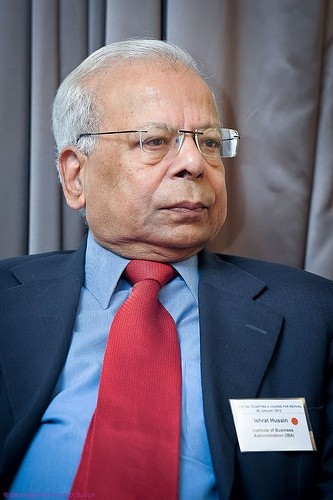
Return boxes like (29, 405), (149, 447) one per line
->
(1, 37), (333, 500)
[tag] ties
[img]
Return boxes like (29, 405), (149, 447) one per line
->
(68, 259), (182, 500)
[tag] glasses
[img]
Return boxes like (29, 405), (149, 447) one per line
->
(75, 124), (240, 158)
